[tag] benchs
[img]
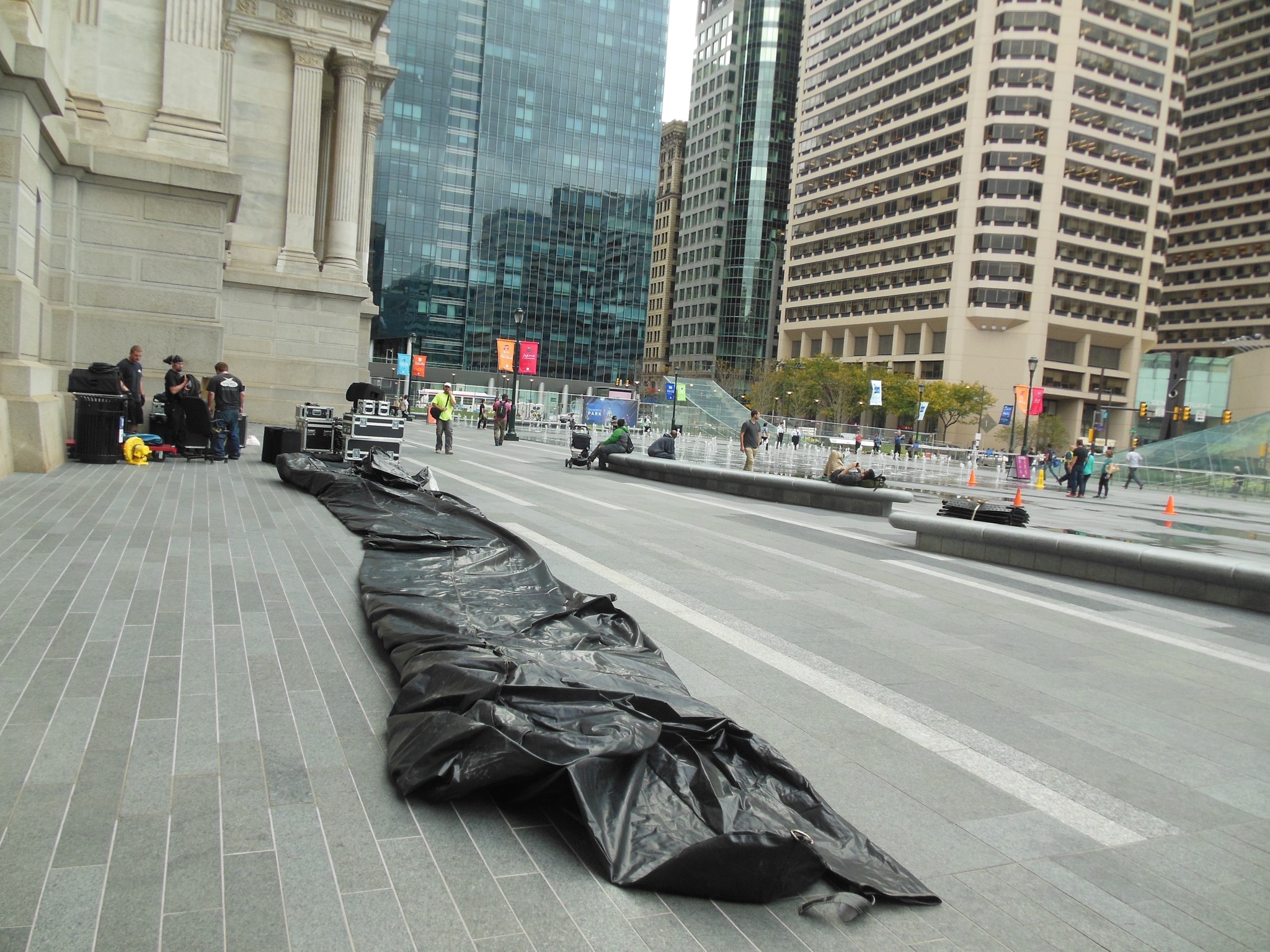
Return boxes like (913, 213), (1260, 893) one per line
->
(888, 512), (1270, 615)
(605, 453), (914, 517)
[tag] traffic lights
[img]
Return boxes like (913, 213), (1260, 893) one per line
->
(1139, 401), (1148, 417)
(626, 379), (630, 387)
(1132, 438), (1138, 449)
(615, 378), (620, 386)
(1183, 406), (1191, 422)
(1221, 410), (1231, 425)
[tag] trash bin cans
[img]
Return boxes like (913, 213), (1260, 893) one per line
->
(675, 425), (682, 436)
(72, 392), (128, 463)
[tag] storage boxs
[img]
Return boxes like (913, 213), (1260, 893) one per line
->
(295, 399), (406, 466)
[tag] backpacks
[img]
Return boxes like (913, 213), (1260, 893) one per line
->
(497, 401), (507, 418)
(859, 474), (886, 488)
(619, 431), (634, 453)
(1048, 449), (1056, 462)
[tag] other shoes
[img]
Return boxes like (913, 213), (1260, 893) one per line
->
(1057, 477), (1108, 499)
(1122, 485), (1127, 489)
(1140, 484), (1143, 490)
(590, 459), (593, 462)
(436, 450), (441, 453)
(181, 451), (194, 457)
(595, 467), (606, 470)
(445, 450), (453, 454)
(499, 443), (502, 446)
(477, 428), (482, 430)
(229, 455), (239, 459)
(495, 439), (499, 446)
(482, 428), (488, 430)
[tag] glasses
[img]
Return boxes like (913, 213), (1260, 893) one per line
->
(864, 469), (866, 475)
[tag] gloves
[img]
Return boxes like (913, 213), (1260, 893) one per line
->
(125, 390), (133, 401)
(141, 395), (145, 406)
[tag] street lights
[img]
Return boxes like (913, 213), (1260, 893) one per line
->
(865, 364), (893, 425)
(913, 382), (925, 459)
(529, 379), (535, 418)
(785, 391), (794, 433)
(774, 397), (780, 426)
(406, 332), (418, 421)
(1020, 355), (1039, 455)
(501, 374), (507, 395)
(857, 400), (865, 425)
(740, 395), (746, 406)
(1093, 387), (1112, 454)
(634, 381), (641, 400)
(671, 365), (680, 431)
(452, 373), (456, 391)
(813, 399), (820, 436)
(966, 385), (986, 450)
(506, 378), (510, 393)
(390, 367), (396, 395)
(503, 308), (525, 441)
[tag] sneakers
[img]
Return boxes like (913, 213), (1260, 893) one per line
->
(842, 451), (850, 463)
(814, 473), (829, 481)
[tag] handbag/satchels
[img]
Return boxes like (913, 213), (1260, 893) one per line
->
(430, 407), (442, 419)
(763, 427), (770, 438)
(1105, 463), (1120, 474)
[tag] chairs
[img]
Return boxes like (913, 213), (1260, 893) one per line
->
(168, 396), (228, 464)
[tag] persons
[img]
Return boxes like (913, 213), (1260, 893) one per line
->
(641, 417), (652, 436)
(476, 399), (489, 430)
(1230, 466), (1244, 494)
(567, 413), (576, 429)
(610, 416), (618, 430)
(967, 447), (971, 456)
(588, 419), (631, 470)
(907, 434), (914, 461)
(163, 356), (194, 458)
(893, 430), (905, 461)
(1028, 447), (1035, 466)
(432, 382), (456, 455)
(493, 393), (512, 446)
(855, 431), (862, 454)
(759, 422), (770, 451)
(1122, 447), (1144, 490)
(813, 450), (875, 486)
(1092, 450), (1115, 500)
(791, 425), (801, 450)
(206, 363), (245, 460)
(872, 431), (882, 455)
(776, 422), (784, 449)
(982, 448), (1007, 455)
(398, 395), (410, 418)
(1058, 439), (1094, 498)
(740, 409), (761, 472)
(1043, 443), (1061, 482)
(1036, 451), (1045, 466)
(647, 429), (678, 461)
(115, 345), (145, 433)
(839, 432), (844, 438)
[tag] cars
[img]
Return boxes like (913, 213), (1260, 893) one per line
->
(637, 417), (651, 424)
(938, 444), (960, 448)
(560, 412), (583, 423)
(837, 432), (856, 439)
(967, 450), (1009, 466)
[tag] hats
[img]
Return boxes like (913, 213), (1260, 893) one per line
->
(780, 422), (784, 424)
(444, 382), (451, 387)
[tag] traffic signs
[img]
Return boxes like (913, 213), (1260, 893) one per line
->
(1194, 409), (1206, 422)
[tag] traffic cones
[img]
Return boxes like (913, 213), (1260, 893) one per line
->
(966, 469), (978, 484)
(1010, 487), (1025, 508)
(1162, 496), (1178, 515)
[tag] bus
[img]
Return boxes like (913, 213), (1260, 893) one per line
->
(414, 388), (502, 414)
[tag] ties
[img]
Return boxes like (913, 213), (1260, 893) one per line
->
(796, 429), (798, 436)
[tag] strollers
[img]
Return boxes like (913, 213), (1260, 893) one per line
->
(565, 425), (591, 470)
